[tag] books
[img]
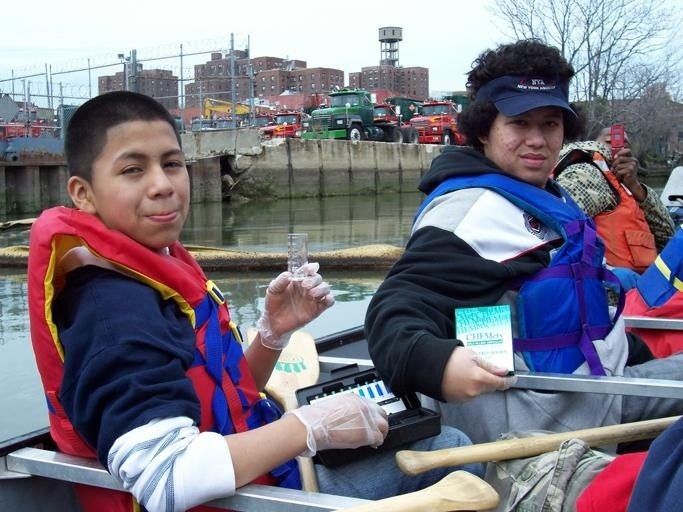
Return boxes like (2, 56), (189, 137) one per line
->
(454, 303), (516, 376)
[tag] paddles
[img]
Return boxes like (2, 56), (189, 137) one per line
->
(248, 327), (320, 492)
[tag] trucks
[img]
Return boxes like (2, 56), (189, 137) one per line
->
(188, 98), (272, 131)
(371, 97), (423, 145)
(302, 90), (383, 142)
(409, 98), (469, 149)
(261, 110), (310, 142)
(0, 102), (78, 139)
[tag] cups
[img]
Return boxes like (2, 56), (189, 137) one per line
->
(286, 232), (310, 282)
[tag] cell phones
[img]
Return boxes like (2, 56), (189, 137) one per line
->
(611, 122), (624, 161)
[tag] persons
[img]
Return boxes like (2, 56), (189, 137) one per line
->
(550, 122), (683, 360)
(22, 91), (482, 512)
(364, 36), (683, 512)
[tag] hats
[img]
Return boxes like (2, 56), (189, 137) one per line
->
(474, 76), (579, 122)
